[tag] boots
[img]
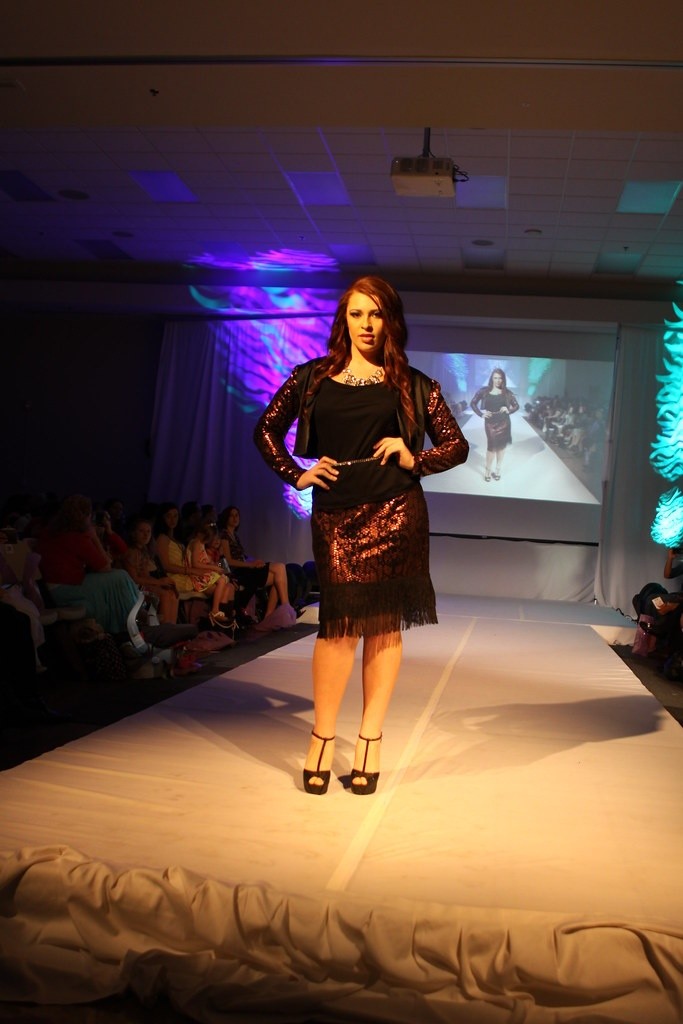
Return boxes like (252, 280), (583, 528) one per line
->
(629, 614), (657, 660)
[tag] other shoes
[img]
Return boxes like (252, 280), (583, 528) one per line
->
(208, 611), (228, 622)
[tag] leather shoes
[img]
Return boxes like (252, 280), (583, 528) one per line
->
(640, 620), (664, 639)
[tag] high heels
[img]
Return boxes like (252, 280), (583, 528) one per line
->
(486, 472), (500, 482)
(351, 733), (382, 793)
(302, 732), (335, 794)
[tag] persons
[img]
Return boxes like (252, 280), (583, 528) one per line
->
(251, 276), (471, 797)
(464, 368), (520, 482)
(634, 540), (683, 684)
(527, 392), (609, 491)
(1, 491), (285, 686)
